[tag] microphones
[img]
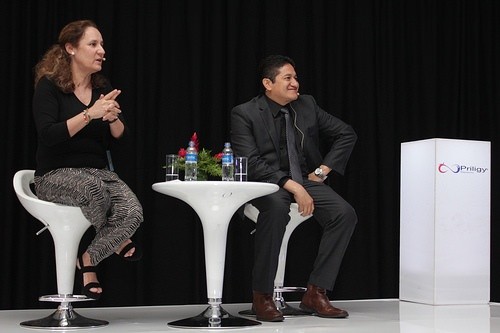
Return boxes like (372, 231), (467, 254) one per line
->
(296, 93), (300, 95)
(102, 58), (106, 61)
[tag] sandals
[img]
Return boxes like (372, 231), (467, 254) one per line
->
(115, 240), (138, 260)
(76, 255), (103, 299)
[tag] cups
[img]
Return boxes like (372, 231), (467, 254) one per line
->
(165, 154), (180, 181)
(234, 157), (248, 181)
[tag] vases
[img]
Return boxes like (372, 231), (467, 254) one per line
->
(197, 170), (208, 181)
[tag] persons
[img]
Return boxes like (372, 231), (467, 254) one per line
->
(230, 56), (357, 322)
(31, 20), (143, 300)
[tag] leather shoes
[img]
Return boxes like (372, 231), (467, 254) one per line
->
(252, 289), (284, 321)
(299, 284), (349, 317)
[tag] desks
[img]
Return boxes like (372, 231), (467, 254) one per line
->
(152, 181), (279, 328)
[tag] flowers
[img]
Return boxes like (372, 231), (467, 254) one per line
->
(175, 132), (223, 177)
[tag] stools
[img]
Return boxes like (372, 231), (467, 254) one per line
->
(13, 169), (111, 330)
(239, 203), (315, 315)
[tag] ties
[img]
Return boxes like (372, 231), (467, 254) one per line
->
(281, 107), (303, 185)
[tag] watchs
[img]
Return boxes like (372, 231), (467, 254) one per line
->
(314, 168), (326, 180)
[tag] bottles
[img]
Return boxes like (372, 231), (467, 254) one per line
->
(184, 141), (198, 181)
(221, 143), (235, 181)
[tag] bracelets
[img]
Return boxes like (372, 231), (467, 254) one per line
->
(82, 108), (91, 124)
(109, 118), (117, 123)
